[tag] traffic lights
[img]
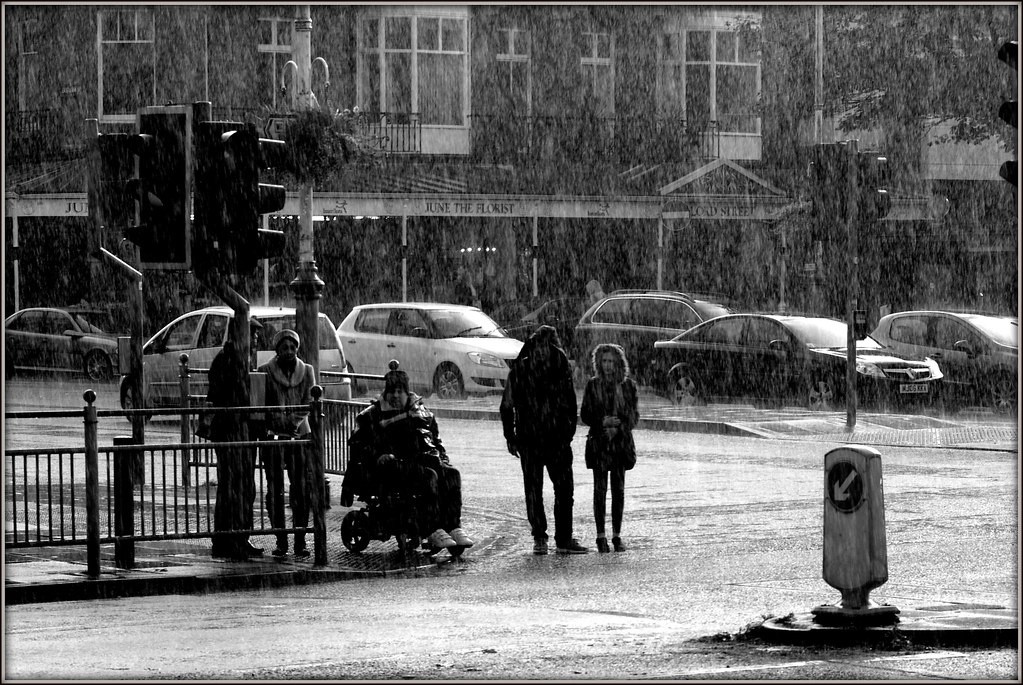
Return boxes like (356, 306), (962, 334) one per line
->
(994, 40), (1018, 187)
(858, 148), (893, 222)
(94, 130), (140, 227)
(123, 105), (193, 272)
(229, 123), (289, 276)
(191, 119), (256, 280)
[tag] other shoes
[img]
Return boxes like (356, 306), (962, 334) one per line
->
(533, 539), (548, 553)
(245, 540), (264, 555)
(556, 539), (588, 553)
(596, 537), (610, 553)
(272, 548), (286, 556)
(294, 548), (311, 555)
(213, 548), (248, 563)
(612, 537), (626, 552)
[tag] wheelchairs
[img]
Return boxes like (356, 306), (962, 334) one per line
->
(339, 427), (465, 558)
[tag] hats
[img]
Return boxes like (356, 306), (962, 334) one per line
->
(273, 329), (300, 350)
(250, 318), (263, 329)
(384, 370), (409, 400)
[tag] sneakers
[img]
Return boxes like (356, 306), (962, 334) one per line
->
(430, 529), (457, 547)
(449, 528), (474, 547)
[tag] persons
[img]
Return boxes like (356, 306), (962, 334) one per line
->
(204, 314), (266, 561)
(358, 370), (475, 549)
(581, 342), (640, 554)
(456, 271), (479, 308)
(498, 326), (590, 554)
(258, 329), (318, 556)
(586, 280), (607, 305)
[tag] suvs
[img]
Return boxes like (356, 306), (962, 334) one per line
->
(570, 287), (757, 391)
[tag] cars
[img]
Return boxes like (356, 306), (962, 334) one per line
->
(486, 295), (592, 361)
(868, 308), (1017, 416)
(651, 311), (945, 410)
(4, 305), (133, 383)
(119, 303), (354, 428)
(335, 301), (526, 400)
